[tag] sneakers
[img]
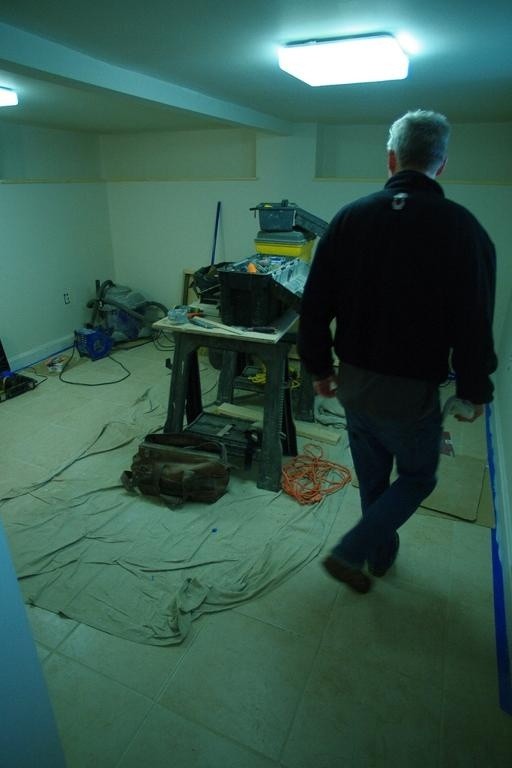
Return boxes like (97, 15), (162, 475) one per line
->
(324, 557), (369, 594)
(369, 530), (398, 577)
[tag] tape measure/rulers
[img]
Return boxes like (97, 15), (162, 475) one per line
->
(193, 316), (243, 335)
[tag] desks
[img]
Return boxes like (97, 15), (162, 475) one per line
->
(147, 286), (309, 495)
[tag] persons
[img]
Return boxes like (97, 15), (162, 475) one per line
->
(298, 108), (500, 593)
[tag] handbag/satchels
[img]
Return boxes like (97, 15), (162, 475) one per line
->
(131, 433), (230, 503)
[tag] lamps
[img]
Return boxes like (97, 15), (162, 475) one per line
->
(274, 29), (412, 89)
